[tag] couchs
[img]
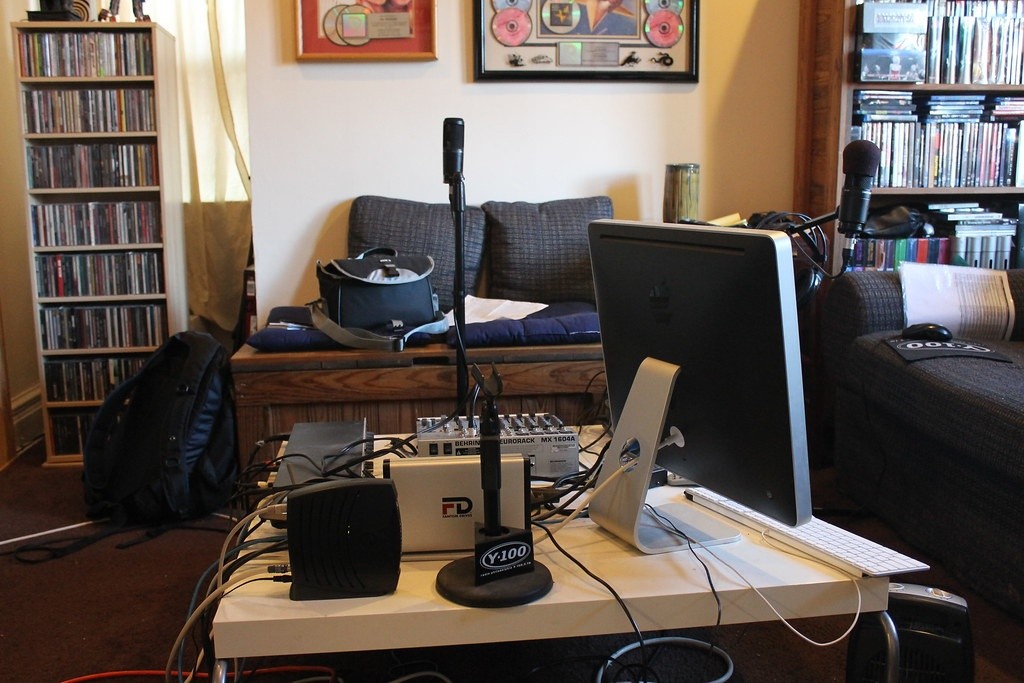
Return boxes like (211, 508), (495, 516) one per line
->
(814, 267), (1024, 628)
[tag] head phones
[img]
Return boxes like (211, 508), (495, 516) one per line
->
(751, 213), (827, 305)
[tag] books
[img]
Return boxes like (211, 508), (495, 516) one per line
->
(40, 303), (164, 350)
(854, 0), (1024, 85)
(845, 198), (1024, 271)
(49, 409), (97, 455)
(18, 30), (154, 77)
(22, 88), (156, 133)
(26, 141), (159, 189)
(851, 89), (1024, 188)
(30, 201), (162, 246)
(34, 251), (165, 298)
(43, 355), (150, 402)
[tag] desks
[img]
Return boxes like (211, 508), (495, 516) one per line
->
(212, 424), (890, 683)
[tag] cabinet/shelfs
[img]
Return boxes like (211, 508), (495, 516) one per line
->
(10, 21), (189, 468)
(792, 0), (1024, 269)
(229, 334), (604, 477)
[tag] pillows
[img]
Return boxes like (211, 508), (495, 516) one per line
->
(482, 195), (614, 304)
(348, 194), (486, 312)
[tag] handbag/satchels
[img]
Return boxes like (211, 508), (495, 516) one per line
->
(864, 202), (927, 239)
(309, 249), (449, 352)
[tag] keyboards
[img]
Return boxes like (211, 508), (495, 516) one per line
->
(683, 486), (930, 578)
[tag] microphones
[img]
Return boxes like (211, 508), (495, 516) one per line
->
(443, 118), (464, 207)
(837, 139), (881, 271)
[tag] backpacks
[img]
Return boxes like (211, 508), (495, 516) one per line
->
(85, 330), (241, 528)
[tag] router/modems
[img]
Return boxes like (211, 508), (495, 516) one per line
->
(285, 479), (402, 601)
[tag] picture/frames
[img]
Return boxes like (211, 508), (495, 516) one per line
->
(295, 0), (435, 60)
(475, 0), (699, 84)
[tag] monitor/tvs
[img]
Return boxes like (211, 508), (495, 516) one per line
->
(587, 218), (813, 555)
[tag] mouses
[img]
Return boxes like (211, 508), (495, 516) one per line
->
(902, 323), (953, 343)
